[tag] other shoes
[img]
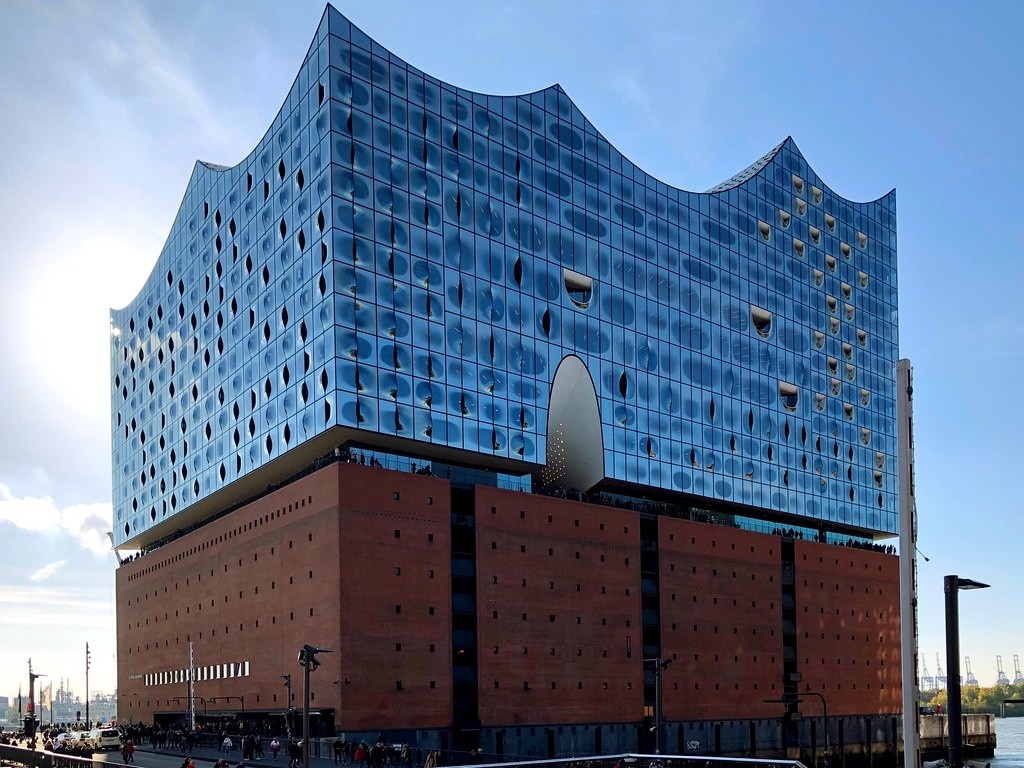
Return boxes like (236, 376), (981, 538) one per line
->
(153, 747), (187, 753)
(228, 754), (231, 756)
(342, 762), (344, 764)
(345, 763), (347, 766)
(225, 753), (227, 756)
(340, 763), (342, 766)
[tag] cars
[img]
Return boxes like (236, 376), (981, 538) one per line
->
(52, 733), (72, 745)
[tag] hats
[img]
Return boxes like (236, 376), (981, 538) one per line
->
(127, 740), (131, 743)
(377, 743), (381, 747)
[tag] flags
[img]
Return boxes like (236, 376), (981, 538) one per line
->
(43, 683), (51, 711)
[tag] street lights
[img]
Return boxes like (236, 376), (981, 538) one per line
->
(172, 696), (207, 735)
(763, 693), (828, 768)
(281, 674), (291, 711)
(207, 696), (246, 737)
(298, 646), (335, 768)
(642, 658), (673, 758)
(944, 575), (991, 768)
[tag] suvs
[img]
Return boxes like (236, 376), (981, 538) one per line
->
(84, 728), (120, 752)
(72, 731), (90, 747)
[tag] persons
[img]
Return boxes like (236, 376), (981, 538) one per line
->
(0, 720), (94, 758)
(123, 720), (243, 753)
(241, 735), (265, 760)
(288, 736), (304, 768)
(270, 737), (281, 760)
(424, 748), (485, 768)
(121, 740), (135, 764)
(333, 738), (410, 768)
(181, 756), (245, 768)
